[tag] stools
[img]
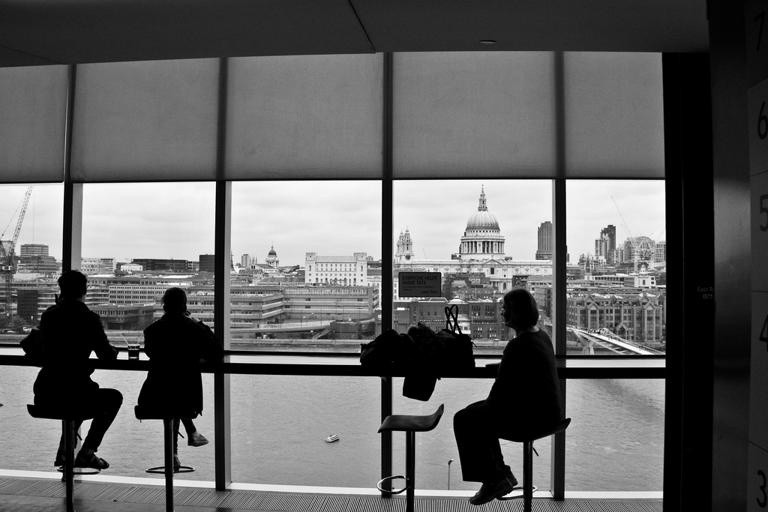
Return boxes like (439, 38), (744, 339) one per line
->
(377, 404), (444, 512)
(134, 400), (198, 512)
(27, 404), (101, 512)
(497, 418), (571, 512)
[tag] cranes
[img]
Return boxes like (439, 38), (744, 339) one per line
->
(0, 186), (35, 321)
(608, 193), (665, 275)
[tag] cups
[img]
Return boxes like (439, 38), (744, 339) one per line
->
(127, 343), (139, 361)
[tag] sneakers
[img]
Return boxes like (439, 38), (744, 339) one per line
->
(470, 468), (518, 505)
(173, 434), (208, 471)
(57, 452), (109, 471)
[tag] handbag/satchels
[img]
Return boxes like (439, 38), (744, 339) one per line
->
(357, 325), (477, 400)
(21, 328), (42, 357)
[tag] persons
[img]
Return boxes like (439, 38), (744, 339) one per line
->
(453, 287), (563, 506)
(31, 270), (124, 469)
(136, 287), (216, 469)
